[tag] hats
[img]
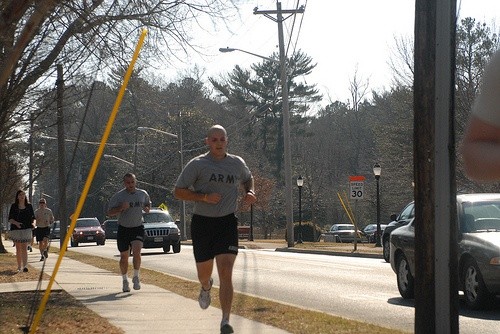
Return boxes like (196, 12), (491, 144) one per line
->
(38, 198), (46, 203)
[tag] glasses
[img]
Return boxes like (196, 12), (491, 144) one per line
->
(39, 202), (46, 204)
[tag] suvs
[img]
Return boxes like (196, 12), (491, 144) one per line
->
(141, 208), (181, 253)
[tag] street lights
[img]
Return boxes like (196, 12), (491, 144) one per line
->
(218, 48), (295, 247)
(104, 154), (137, 177)
(137, 126), (187, 241)
(296, 175), (304, 243)
(373, 162), (382, 246)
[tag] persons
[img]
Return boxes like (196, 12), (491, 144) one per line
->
(462, 49), (500, 185)
(172, 124), (256, 334)
(108, 173), (153, 292)
(8, 190), (37, 273)
(27, 229), (36, 252)
(33, 198), (54, 261)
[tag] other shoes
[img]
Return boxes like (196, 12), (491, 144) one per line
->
(40, 256), (45, 261)
(43, 251), (48, 258)
(22, 266), (28, 273)
(17, 268), (22, 273)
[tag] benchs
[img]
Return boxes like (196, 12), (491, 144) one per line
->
(238, 226), (251, 240)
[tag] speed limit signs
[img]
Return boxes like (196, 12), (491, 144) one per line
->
(350, 181), (363, 199)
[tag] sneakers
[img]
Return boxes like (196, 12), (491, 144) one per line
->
(131, 276), (141, 290)
(219, 319), (233, 334)
(198, 277), (214, 309)
(122, 279), (131, 292)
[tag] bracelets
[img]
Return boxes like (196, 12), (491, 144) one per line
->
(204, 194), (207, 201)
(245, 190), (255, 195)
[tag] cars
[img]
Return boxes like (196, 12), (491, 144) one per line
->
(362, 224), (387, 243)
(50, 221), (61, 239)
(382, 200), (415, 263)
(389, 192), (500, 311)
(323, 224), (362, 243)
(101, 220), (118, 239)
(71, 217), (106, 247)
